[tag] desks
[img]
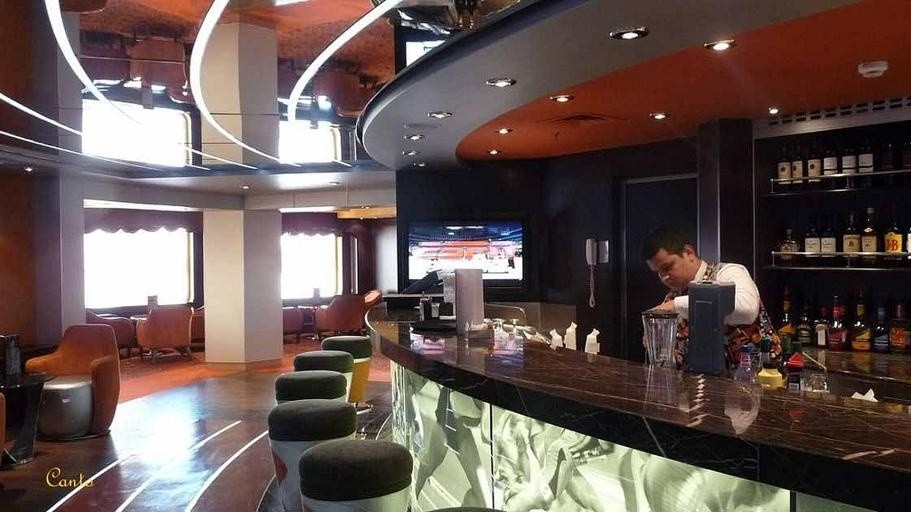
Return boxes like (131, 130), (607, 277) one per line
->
(4, 372), (57, 467)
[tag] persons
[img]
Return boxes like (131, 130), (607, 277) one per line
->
(643, 228), (788, 388)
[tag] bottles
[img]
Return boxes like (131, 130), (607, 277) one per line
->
(773, 287), (911, 356)
(769, 208), (911, 269)
(734, 338), (806, 390)
(759, 137), (911, 190)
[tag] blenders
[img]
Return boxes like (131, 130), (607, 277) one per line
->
(641, 312), (680, 371)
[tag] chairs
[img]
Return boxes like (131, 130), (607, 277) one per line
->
(25, 324), (120, 442)
(79, 22), (373, 118)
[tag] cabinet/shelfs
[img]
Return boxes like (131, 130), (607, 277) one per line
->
(752, 97), (911, 406)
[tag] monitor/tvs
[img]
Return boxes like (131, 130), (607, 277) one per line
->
(406, 216), (530, 293)
